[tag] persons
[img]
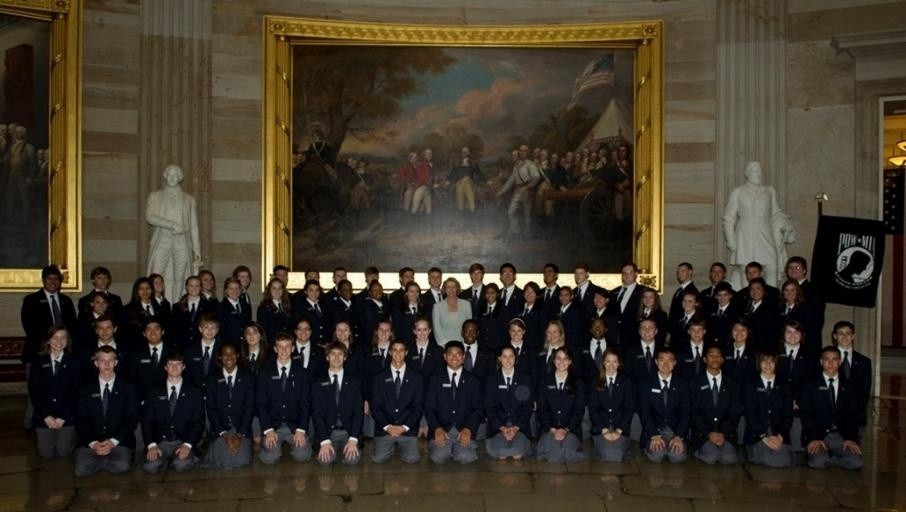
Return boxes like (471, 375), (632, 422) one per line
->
(634, 289), (675, 350)
(293, 279), (327, 342)
(701, 281), (737, 345)
(21, 264), (76, 439)
(122, 276), (167, 342)
(609, 263), (649, 347)
(746, 277), (774, 335)
(333, 320), (367, 372)
(540, 263), (561, 319)
(799, 346), (864, 469)
(361, 320), (397, 437)
(629, 319), (668, 442)
(358, 279), (390, 341)
(460, 320), (492, 440)
(669, 263), (699, 329)
(676, 320), (716, 380)
(838, 250), (870, 284)
(833, 320), (872, 451)
(779, 279), (814, 337)
(443, 147), (492, 216)
(78, 267), (122, 320)
(392, 281), (430, 348)
(289, 320), (326, 440)
(148, 274), (172, 318)
(390, 268), (423, 314)
(204, 345), (255, 469)
(323, 149), (439, 217)
(68, 345), (138, 477)
(552, 286), (581, 342)
(28, 325), (82, 459)
(74, 291), (123, 348)
(405, 319), (444, 439)
(512, 282), (548, 333)
(198, 270), (219, 305)
(257, 279), (293, 346)
(237, 321), (275, 444)
(488, 145), (633, 240)
(172, 276), (211, 354)
(484, 346), (534, 460)
(215, 278), (247, 354)
(688, 345), (740, 464)
(323, 279), (355, 340)
(0, 124), (48, 228)
(459, 263), (486, 320)
(368, 340), (423, 463)
(233, 265), (252, 321)
(701, 263), (736, 321)
(574, 317), (621, 439)
(736, 262), (780, 307)
(325, 265), (347, 299)
(744, 350), (793, 468)
(535, 347), (585, 462)
(723, 161), (796, 290)
(424, 341), (482, 465)
(475, 283), (508, 350)
(144, 164), (204, 307)
(131, 318), (177, 451)
(572, 263), (601, 319)
(787, 256), (825, 359)
(78, 315), (135, 386)
(771, 320), (813, 451)
(672, 291), (704, 339)
(721, 319), (761, 445)
(354, 266), (388, 304)
(588, 349), (632, 463)
(497, 263), (527, 314)
(293, 268), (325, 298)
(274, 265), (292, 305)
(421, 267), (448, 319)
(639, 349), (690, 464)
(490, 317), (533, 376)
(183, 315), (226, 446)
(141, 354), (206, 475)
(586, 289), (617, 348)
(530, 319), (573, 439)
(311, 341), (363, 466)
(253, 333), (313, 464)
(432, 279), (472, 349)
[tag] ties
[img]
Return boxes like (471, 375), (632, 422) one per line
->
(718, 309), (722, 319)
(418, 348), (425, 370)
(680, 316), (688, 331)
(412, 307), (417, 322)
(608, 377), (613, 398)
(694, 346), (701, 375)
(168, 386), (177, 415)
(463, 346), (472, 374)
(577, 288), (581, 301)
(379, 348), (385, 368)
(788, 350), (793, 370)
(202, 346), (211, 375)
(618, 287), (627, 304)
(548, 349), (556, 365)
(501, 289), (508, 306)
(280, 367), (287, 392)
(662, 380), (669, 407)
(735, 350), (740, 365)
(711, 378), (718, 408)
(227, 376), (233, 401)
(506, 377), (511, 392)
(828, 378), (835, 408)
(278, 302), (283, 313)
(750, 306), (755, 313)
(678, 287), (683, 296)
(145, 305), (151, 319)
(190, 302), (195, 323)
(558, 382), (563, 397)
(488, 306), (491, 320)
(766, 381), (771, 399)
(53, 359), (60, 376)
(249, 354), (257, 378)
(516, 347), (520, 355)
(545, 289), (551, 302)
(472, 290), (478, 311)
(394, 371), (401, 399)
(451, 373), (457, 400)
(50, 294), (62, 328)
(151, 347), (158, 366)
(299, 347), (305, 368)
(438, 292), (442, 303)
(786, 307), (792, 317)
(645, 345), (652, 372)
(332, 374), (340, 409)
(594, 341), (603, 371)
(843, 350), (850, 379)
(235, 304), (241, 315)
(522, 308), (529, 323)
(101, 383), (109, 416)
(314, 303), (322, 318)
